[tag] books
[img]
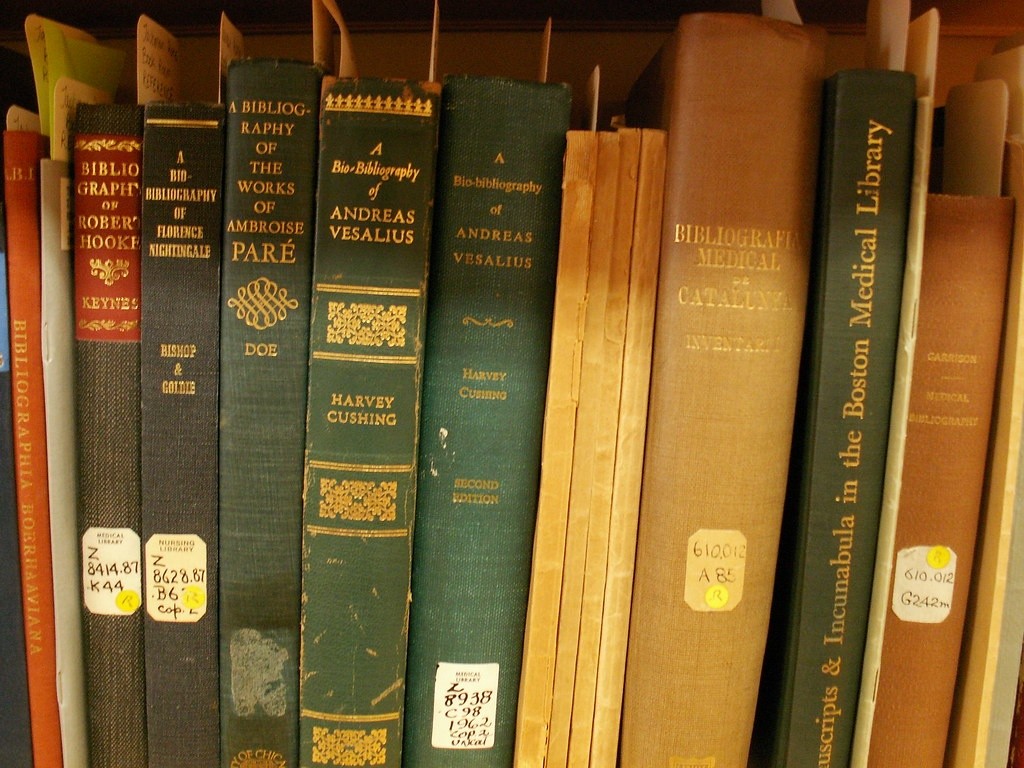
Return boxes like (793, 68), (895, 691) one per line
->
(0, 1), (1023, 768)
